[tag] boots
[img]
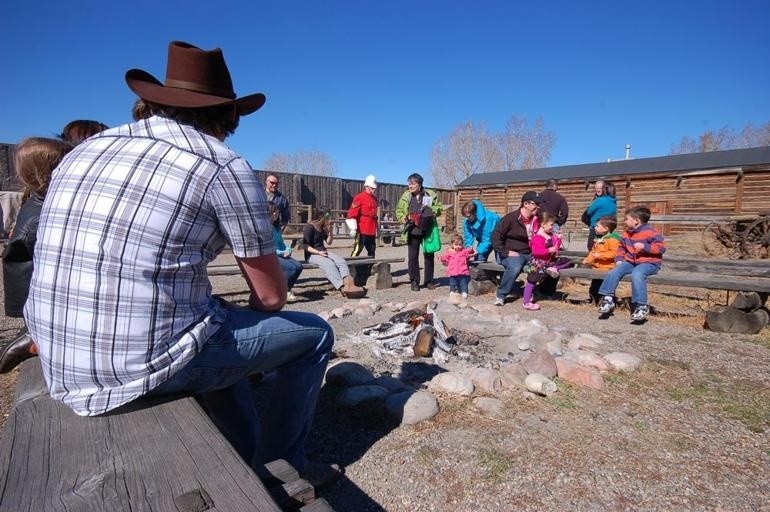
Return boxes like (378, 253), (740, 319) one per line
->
(340, 277), (368, 298)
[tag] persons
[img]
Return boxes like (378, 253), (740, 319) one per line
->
(345, 175), (379, 258)
(0, 137), (76, 376)
(24, 41), (342, 490)
(268, 201), (303, 302)
(264, 174), (290, 226)
(60, 120), (110, 148)
(438, 179), (666, 321)
(303, 207), (366, 295)
(396, 173), (442, 291)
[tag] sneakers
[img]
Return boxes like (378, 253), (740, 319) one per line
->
(427, 283), (435, 290)
(598, 299), (615, 314)
(411, 281), (420, 290)
(545, 266), (559, 278)
(494, 296), (504, 306)
(286, 291), (297, 302)
(631, 306), (649, 321)
(524, 302), (540, 310)
(303, 458), (341, 490)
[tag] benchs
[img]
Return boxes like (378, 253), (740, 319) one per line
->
(0, 342), (338, 512)
(206, 253), (405, 290)
(476, 250), (770, 318)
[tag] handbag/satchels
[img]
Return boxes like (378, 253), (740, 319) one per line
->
(422, 222), (441, 253)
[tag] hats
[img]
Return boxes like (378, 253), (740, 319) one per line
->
(124, 41), (265, 116)
(364, 175), (377, 189)
(521, 190), (547, 204)
